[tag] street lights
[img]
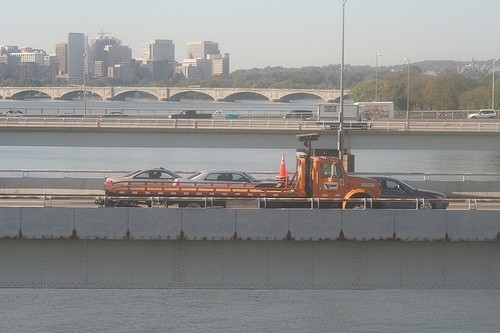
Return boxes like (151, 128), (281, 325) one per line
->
(404, 57), (412, 131)
(375, 53), (383, 100)
(492, 58), (500, 110)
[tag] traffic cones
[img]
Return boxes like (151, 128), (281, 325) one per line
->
(277, 155), (289, 178)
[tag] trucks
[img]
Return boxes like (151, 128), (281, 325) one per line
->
(317, 104), (374, 129)
(103, 155), (380, 210)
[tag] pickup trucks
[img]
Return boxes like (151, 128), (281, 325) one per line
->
(168, 110), (213, 120)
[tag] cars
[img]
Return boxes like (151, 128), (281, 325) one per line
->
(172, 170), (277, 196)
(468, 109), (497, 119)
(103, 167), (183, 193)
(283, 110), (315, 121)
(370, 177), (450, 209)
